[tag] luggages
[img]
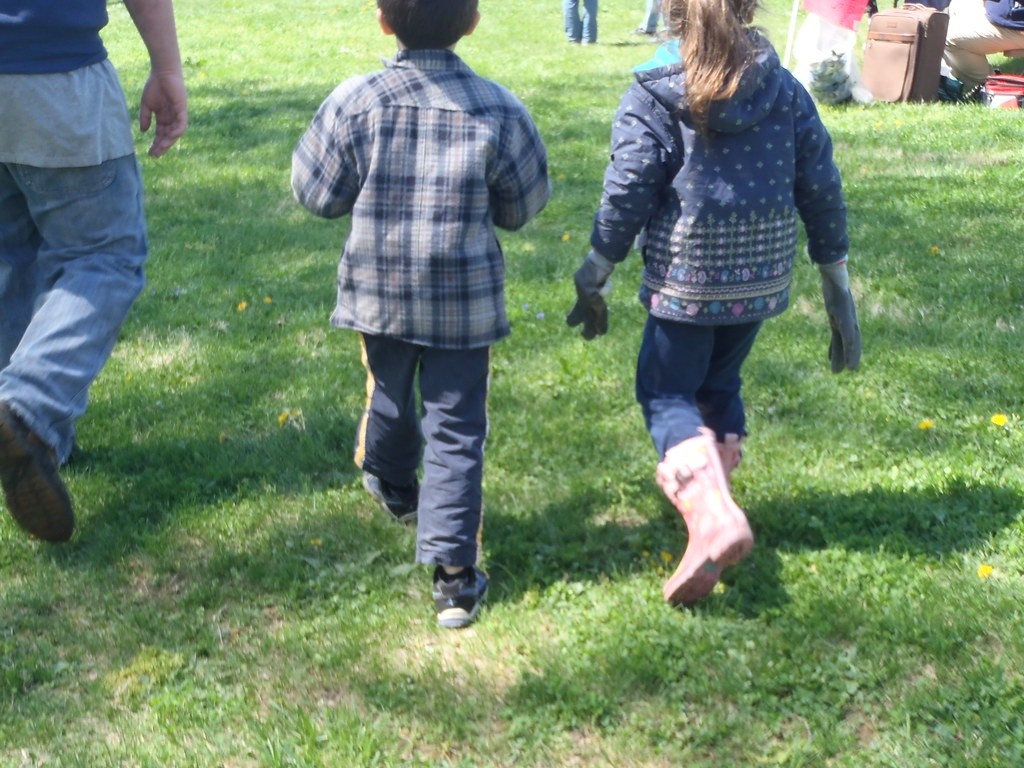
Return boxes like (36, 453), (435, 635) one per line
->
(862, 2), (949, 103)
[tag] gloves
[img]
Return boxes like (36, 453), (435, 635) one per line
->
(818, 255), (862, 375)
(567, 250), (616, 341)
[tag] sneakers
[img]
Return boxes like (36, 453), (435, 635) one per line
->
(433, 566), (489, 628)
(361, 471), (421, 524)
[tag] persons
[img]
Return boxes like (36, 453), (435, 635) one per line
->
(903, 0), (1024, 103)
(290, 0), (551, 628)
(0, 0), (189, 543)
(630, 0), (674, 43)
(563, 0), (601, 46)
(567, 0), (860, 609)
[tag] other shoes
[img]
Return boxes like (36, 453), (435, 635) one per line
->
(1, 401), (74, 544)
(631, 28), (656, 37)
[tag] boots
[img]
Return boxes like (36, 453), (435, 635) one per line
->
(655, 426), (754, 609)
(718, 433), (748, 482)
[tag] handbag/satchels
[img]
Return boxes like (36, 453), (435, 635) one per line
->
(983, 69), (1024, 109)
